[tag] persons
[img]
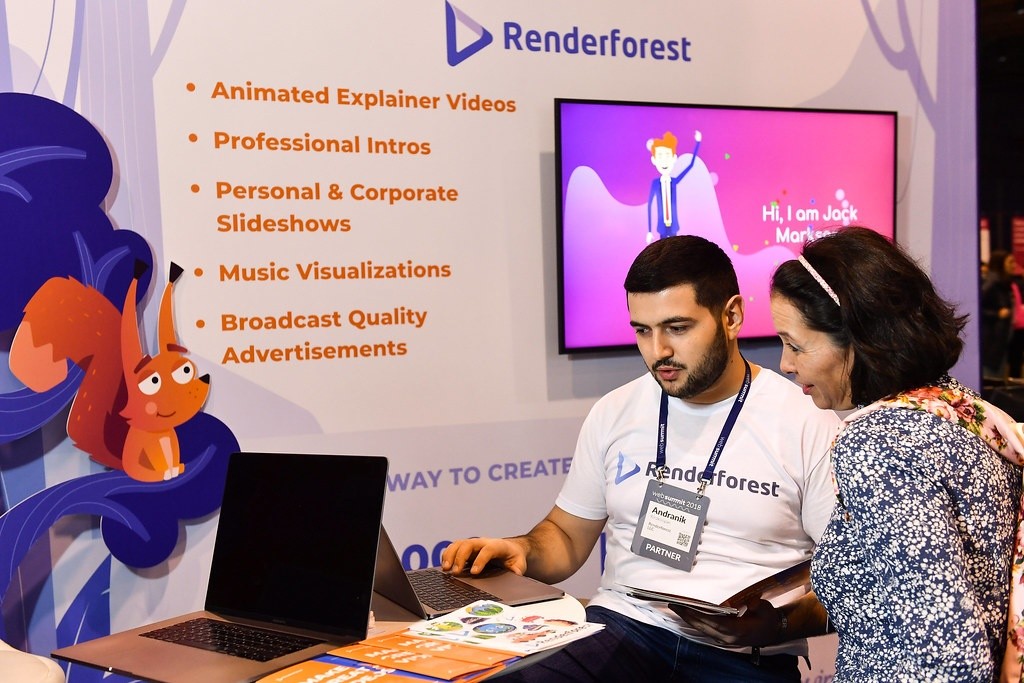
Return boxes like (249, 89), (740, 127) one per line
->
(438, 235), (843, 683)
(772, 221), (1024, 683)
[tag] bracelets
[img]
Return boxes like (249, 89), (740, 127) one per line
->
(775, 608), (788, 643)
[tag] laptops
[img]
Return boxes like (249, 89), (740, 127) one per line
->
(49, 453), (390, 683)
(374, 523), (565, 620)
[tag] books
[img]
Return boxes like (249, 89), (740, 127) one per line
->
(617, 555), (812, 650)
(254, 599), (605, 683)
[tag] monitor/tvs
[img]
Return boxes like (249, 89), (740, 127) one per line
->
(554, 97), (899, 355)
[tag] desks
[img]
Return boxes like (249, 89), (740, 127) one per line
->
(252, 571), (588, 683)
